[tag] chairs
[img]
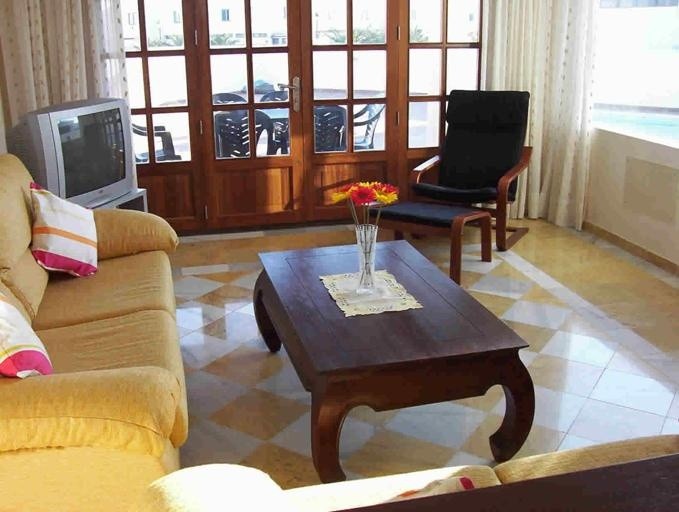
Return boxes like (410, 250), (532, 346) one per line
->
(209, 90), (387, 157)
(132, 123), (180, 162)
(409, 90), (533, 249)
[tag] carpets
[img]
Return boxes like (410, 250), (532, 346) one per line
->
(506, 218), (679, 358)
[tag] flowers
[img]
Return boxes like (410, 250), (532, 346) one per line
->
(324, 182), (401, 286)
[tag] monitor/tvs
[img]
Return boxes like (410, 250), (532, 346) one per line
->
(6, 98), (138, 208)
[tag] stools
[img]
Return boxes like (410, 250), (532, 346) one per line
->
(370, 202), (493, 287)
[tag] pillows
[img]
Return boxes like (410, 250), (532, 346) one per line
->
(28, 183), (99, 277)
(0, 301), (52, 380)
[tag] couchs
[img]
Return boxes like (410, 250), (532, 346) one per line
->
(0, 149), (189, 512)
(149, 433), (679, 512)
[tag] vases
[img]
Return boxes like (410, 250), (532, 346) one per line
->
(356, 224), (380, 286)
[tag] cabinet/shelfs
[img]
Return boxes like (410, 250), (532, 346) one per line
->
(106, 188), (149, 213)
